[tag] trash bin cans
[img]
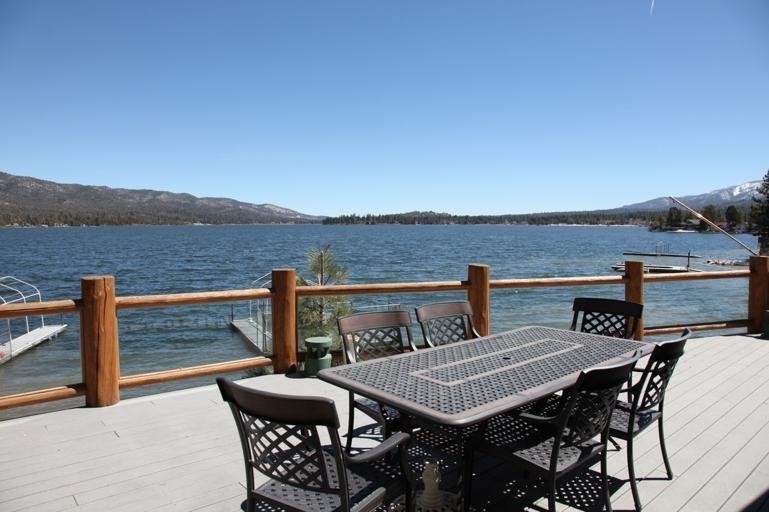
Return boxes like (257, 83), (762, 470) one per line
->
(305, 336), (333, 377)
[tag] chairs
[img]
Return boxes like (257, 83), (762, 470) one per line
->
(466, 347), (641, 511)
(214, 378), (413, 511)
(534, 298), (644, 417)
(574, 328), (692, 511)
(412, 302), (489, 440)
(336, 311), (418, 457)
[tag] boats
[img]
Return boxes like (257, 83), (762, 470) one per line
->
(622, 251), (702, 258)
(611, 261), (688, 272)
(668, 229), (695, 233)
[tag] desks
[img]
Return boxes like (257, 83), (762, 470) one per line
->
(318, 325), (658, 510)
